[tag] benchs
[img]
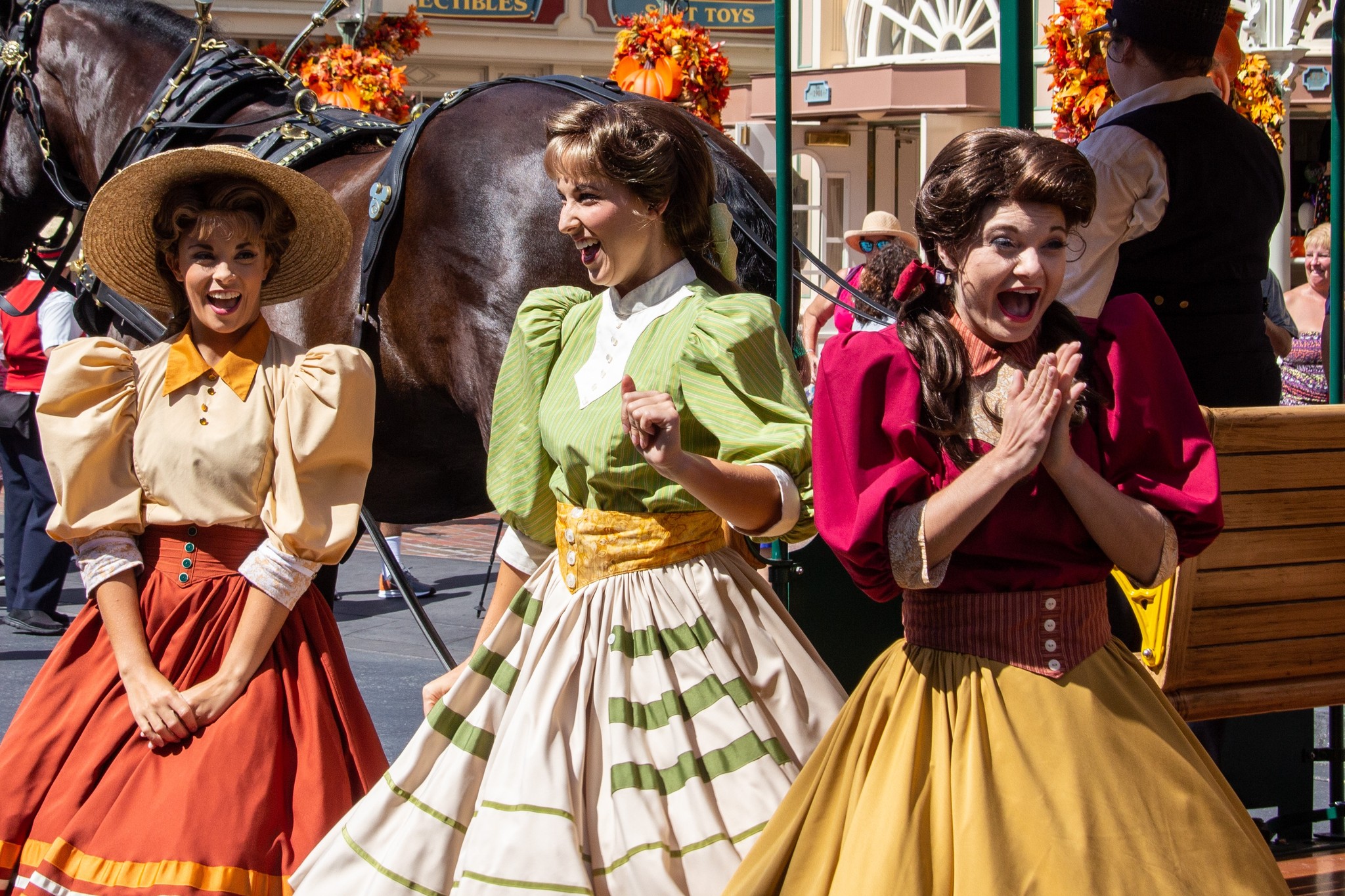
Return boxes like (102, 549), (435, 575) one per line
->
(1105, 393), (1345, 733)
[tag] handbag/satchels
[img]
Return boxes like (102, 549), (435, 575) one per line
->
(0, 389), (40, 444)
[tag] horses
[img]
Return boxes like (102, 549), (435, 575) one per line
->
(0, 1), (800, 525)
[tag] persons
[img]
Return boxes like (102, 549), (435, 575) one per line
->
(378, 520), (437, 599)
(799, 210), (919, 386)
(288, 97), (849, 896)
(0, 142), (390, 895)
(723, 124), (1290, 894)
(1260, 267), (1299, 362)
(1320, 285), (1331, 388)
(0, 217), (89, 634)
(1298, 202), (1315, 238)
(851, 241), (925, 332)
(1276, 221), (1331, 406)
(1053, 0), (1285, 409)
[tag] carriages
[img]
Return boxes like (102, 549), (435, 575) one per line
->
(2, 1), (1345, 894)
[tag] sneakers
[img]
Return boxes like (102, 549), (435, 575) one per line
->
(2, 605), (70, 634)
(378, 565), (436, 598)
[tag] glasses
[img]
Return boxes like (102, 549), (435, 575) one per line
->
(858, 238), (892, 253)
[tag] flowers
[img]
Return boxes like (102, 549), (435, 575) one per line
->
(606, 8), (735, 146)
(1033, 0), (1294, 161)
(251, 3), (433, 129)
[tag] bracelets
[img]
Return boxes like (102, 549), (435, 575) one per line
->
(805, 349), (815, 353)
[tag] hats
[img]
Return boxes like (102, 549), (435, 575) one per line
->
(82, 144), (354, 309)
(844, 210), (919, 255)
(1086, 0), (1231, 58)
(21, 216), (85, 269)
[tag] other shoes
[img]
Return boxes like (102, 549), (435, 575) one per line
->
(0, 559), (7, 584)
